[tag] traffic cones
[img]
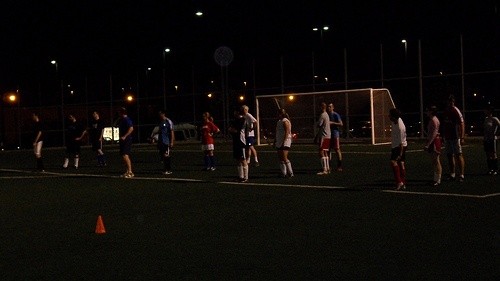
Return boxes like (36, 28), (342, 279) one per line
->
(93, 215), (106, 235)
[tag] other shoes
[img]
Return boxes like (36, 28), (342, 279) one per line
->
(432, 176), (440, 185)
(459, 174), (464, 182)
(239, 177), (248, 183)
(449, 173), (456, 178)
(162, 169), (172, 175)
(392, 181), (406, 190)
(316, 170), (331, 175)
(119, 171), (134, 179)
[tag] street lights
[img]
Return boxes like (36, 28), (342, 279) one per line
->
(401, 40), (408, 67)
(162, 49), (170, 112)
(312, 26), (329, 48)
(51, 60), (58, 72)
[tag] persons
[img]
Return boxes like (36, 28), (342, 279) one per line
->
(155, 110), (175, 174)
(60, 113), (87, 168)
(30, 110), (45, 172)
(87, 109), (107, 167)
(200, 111), (220, 171)
(440, 94), (466, 182)
(228, 105), (259, 183)
(423, 110), (442, 186)
(315, 100), (344, 175)
(114, 106), (135, 178)
(273, 109), (295, 176)
(482, 106), (500, 175)
(388, 109), (408, 190)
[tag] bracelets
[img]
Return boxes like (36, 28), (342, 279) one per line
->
(461, 138), (464, 139)
